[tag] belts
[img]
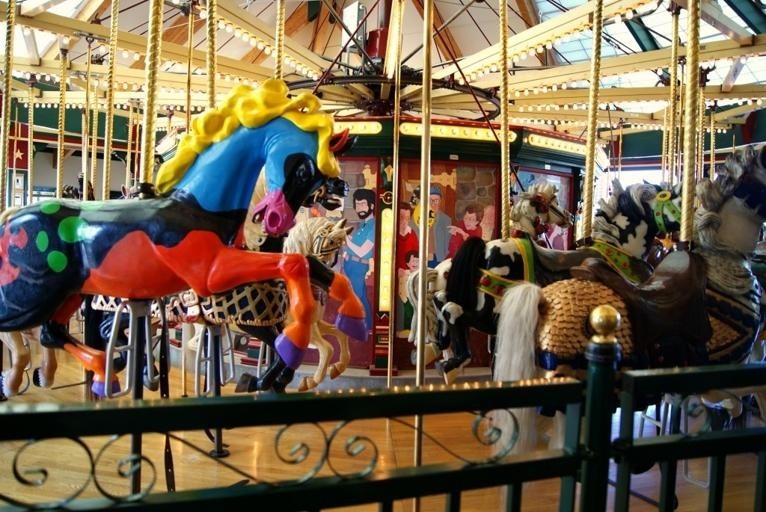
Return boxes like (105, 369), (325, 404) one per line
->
(344, 254), (369, 265)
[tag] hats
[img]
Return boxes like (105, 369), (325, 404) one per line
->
(430, 187), (442, 195)
